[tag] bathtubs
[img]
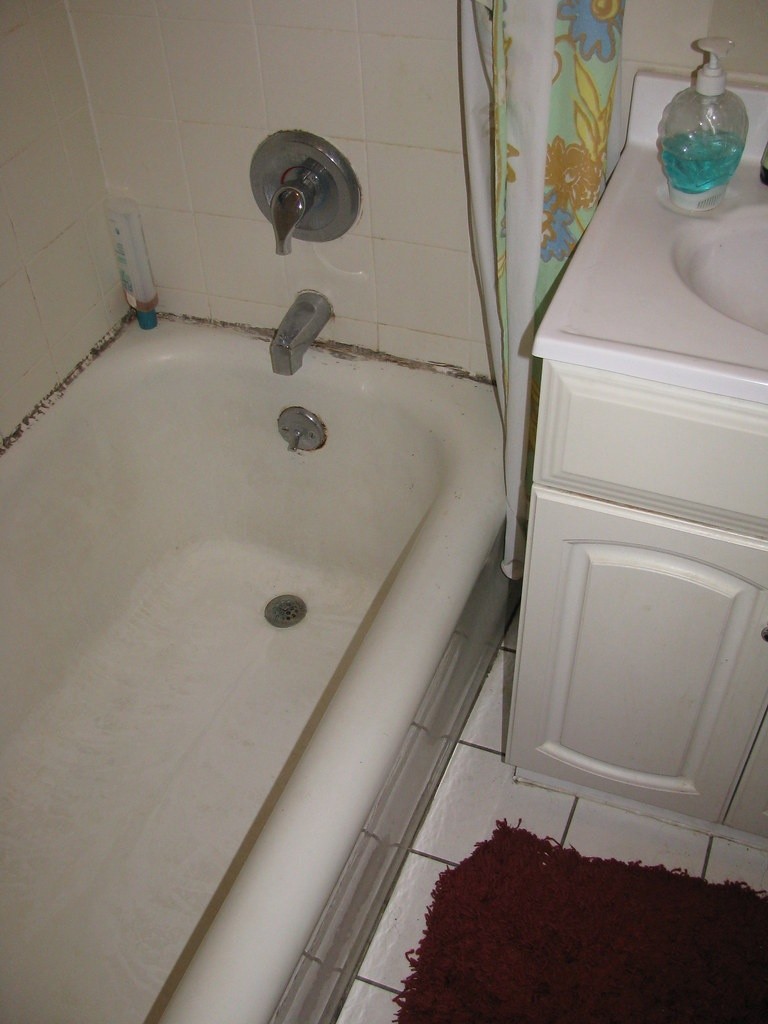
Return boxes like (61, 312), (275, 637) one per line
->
(0, 313), (512, 1023)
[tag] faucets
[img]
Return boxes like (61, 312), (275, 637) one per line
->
(269, 292), (333, 375)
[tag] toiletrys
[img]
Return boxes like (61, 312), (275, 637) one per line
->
(661, 38), (750, 212)
(103, 195), (159, 330)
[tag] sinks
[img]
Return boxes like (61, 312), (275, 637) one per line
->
(670, 205), (767, 334)
